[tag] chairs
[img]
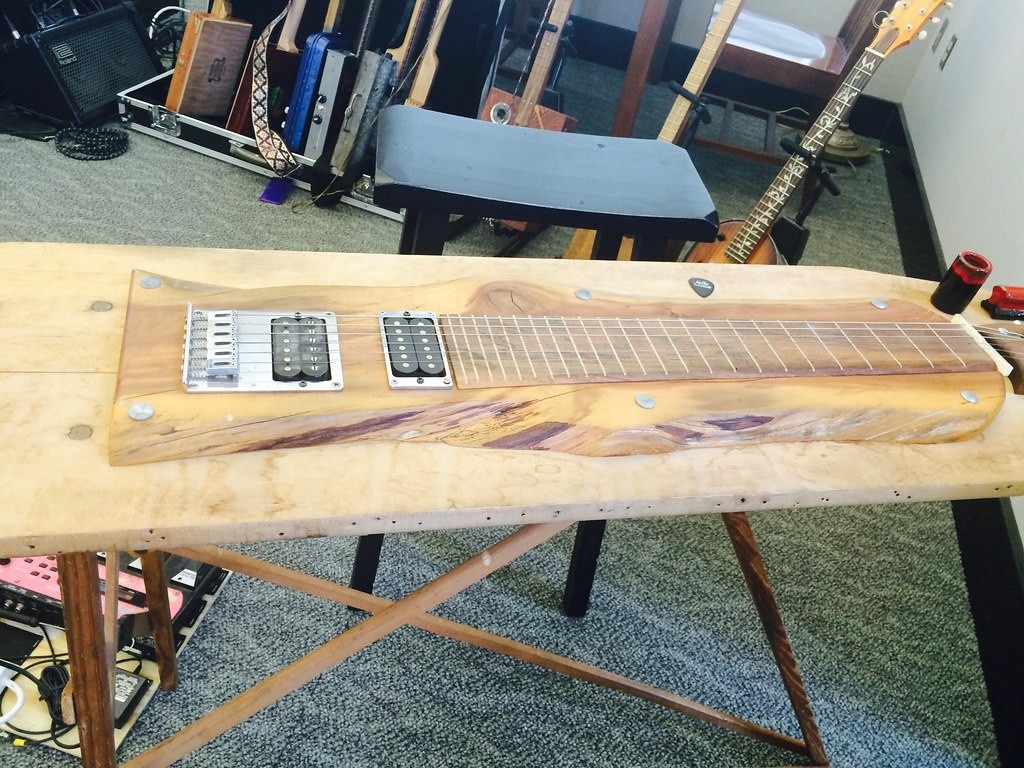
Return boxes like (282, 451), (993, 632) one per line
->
(681, 0), (899, 217)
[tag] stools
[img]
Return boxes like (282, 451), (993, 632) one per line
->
(349, 104), (717, 619)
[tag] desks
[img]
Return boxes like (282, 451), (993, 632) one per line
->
(1, 238), (1024, 768)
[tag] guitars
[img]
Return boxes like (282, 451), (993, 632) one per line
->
(163, 0), (745, 266)
(101, 265), (1024, 473)
(681, 0), (956, 272)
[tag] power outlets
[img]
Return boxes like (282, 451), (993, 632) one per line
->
(931, 18), (948, 52)
(939, 35), (957, 68)
